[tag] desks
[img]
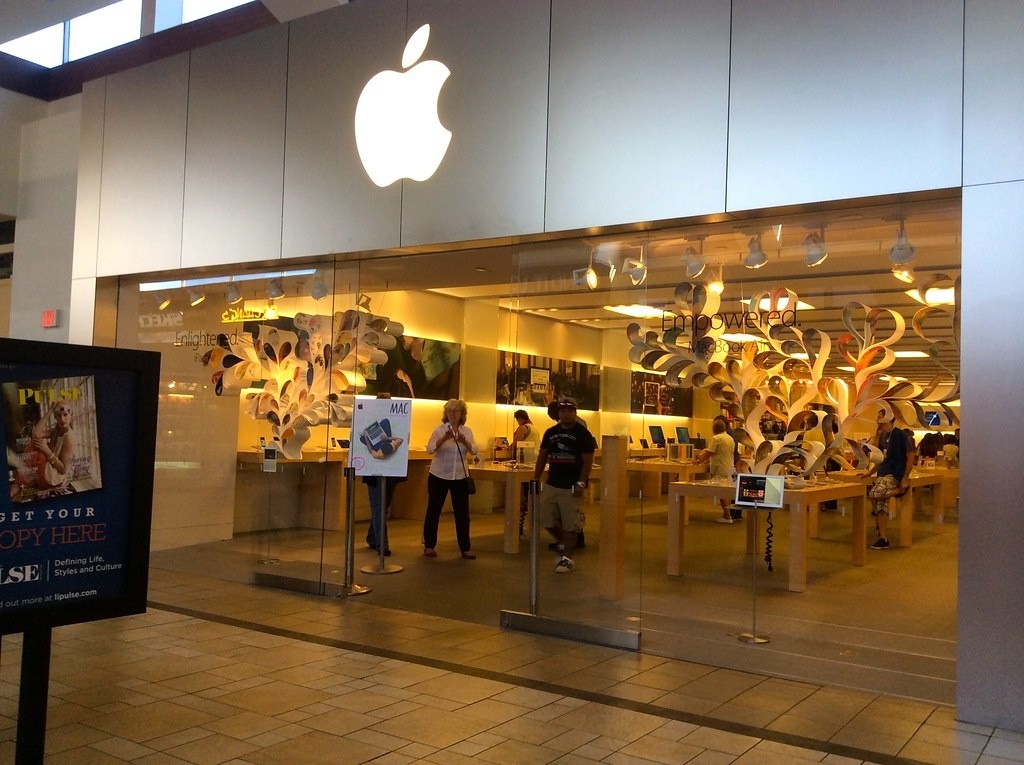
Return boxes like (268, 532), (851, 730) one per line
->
(238, 447), (958, 593)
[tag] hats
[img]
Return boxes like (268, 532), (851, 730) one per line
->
(558, 397), (577, 409)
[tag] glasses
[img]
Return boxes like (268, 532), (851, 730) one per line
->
(560, 402), (573, 407)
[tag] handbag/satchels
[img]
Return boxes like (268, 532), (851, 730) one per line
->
(467, 478), (475, 494)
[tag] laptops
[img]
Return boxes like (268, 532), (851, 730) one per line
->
(364, 421), (387, 446)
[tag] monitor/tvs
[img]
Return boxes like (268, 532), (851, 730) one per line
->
(649, 426), (665, 443)
(677, 427), (691, 444)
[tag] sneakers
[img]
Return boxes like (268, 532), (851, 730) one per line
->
(871, 538), (889, 548)
(894, 484), (909, 498)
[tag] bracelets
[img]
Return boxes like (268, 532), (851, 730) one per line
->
(48, 454), (58, 464)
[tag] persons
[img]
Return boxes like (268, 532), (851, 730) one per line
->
(364, 432), (403, 460)
(770, 412), (959, 515)
(423, 399), (477, 559)
(361, 391), (409, 557)
(29, 399), (76, 493)
(691, 414), (743, 524)
(510, 410), (541, 459)
(861, 409), (914, 550)
(533, 398), (594, 574)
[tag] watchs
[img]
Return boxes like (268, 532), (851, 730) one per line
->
(577, 481), (586, 487)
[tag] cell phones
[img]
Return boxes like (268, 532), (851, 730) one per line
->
(332, 438), (336, 447)
(261, 437), (265, 447)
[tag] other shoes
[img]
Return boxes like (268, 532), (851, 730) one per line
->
(577, 532), (585, 547)
(549, 542), (560, 549)
(557, 543), (565, 551)
(554, 556), (575, 573)
(424, 548), (436, 556)
(462, 551), (476, 559)
(732, 515), (742, 521)
(385, 548), (391, 556)
(366, 539), (376, 548)
(716, 517), (733, 524)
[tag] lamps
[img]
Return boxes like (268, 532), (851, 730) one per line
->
(573, 250), (597, 288)
(226, 282), (242, 304)
(801, 223), (828, 268)
(744, 233), (767, 269)
(153, 291), (171, 311)
(889, 209), (913, 264)
(681, 239), (705, 277)
(267, 278), (285, 299)
(891, 255), (915, 283)
(358, 293), (371, 313)
(186, 286), (206, 306)
(311, 269), (328, 299)
(622, 243), (647, 285)
(707, 264), (724, 294)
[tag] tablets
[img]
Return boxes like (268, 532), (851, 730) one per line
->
(644, 381), (660, 407)
(529, 366), (551, 393)
(640, 439), (649, 448)
